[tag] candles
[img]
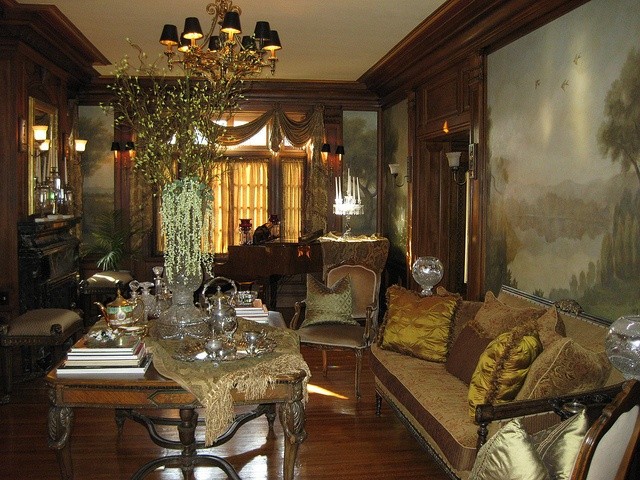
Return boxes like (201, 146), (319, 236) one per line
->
(334, 168), (360, 205)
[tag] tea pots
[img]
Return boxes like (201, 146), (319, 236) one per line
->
(193, 276), (238, 332)
(93, 288), (144, 328)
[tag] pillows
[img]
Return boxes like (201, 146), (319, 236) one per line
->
(445, 290), (547, 388)
(469, 417), (552, 480)
(469, 321), (544, 420)
(537, 307), (565, 348)
(511, 338), (613, 402)
(537, 408), (591, 480)
(301, 273), (358, 327)
(377, 285), (462, 362)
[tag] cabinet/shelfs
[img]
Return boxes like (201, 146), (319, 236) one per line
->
(228, 241), (323, 308)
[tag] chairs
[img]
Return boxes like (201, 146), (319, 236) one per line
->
(289, 257), (385, 398)
(572, 380), (639, 479)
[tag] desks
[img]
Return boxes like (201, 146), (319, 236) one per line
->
(42, 310), (307, 479)
(314, 231), (390, 289)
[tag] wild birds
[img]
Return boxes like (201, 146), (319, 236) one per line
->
(573, 52), (582, 66)
(534, 79), (540, 90)
(560, 77), (569, 91)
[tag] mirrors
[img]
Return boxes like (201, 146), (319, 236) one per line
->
(29, 95), (61, 215)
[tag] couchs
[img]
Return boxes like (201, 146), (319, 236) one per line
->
(367, 284), (639, 480)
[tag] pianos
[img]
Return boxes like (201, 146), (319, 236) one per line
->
(213, 240), (322, 312)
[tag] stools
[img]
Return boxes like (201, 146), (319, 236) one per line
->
(75, 270), (134, 318)
(0, 307), (85, 405)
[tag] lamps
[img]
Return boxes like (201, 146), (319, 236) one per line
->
(335, 145), (344, 160)
(321, 144), (330, 162)
(69, 138), (87, 164)
(605, 316), (640, 379)
(17, 117), (49, 157)
(412, 256), (444, 297)
(389, 164), (407, 188)
(445, 152), (466, 185)
(110, 141), (135, 152)
(159, 0), (282, 90)
